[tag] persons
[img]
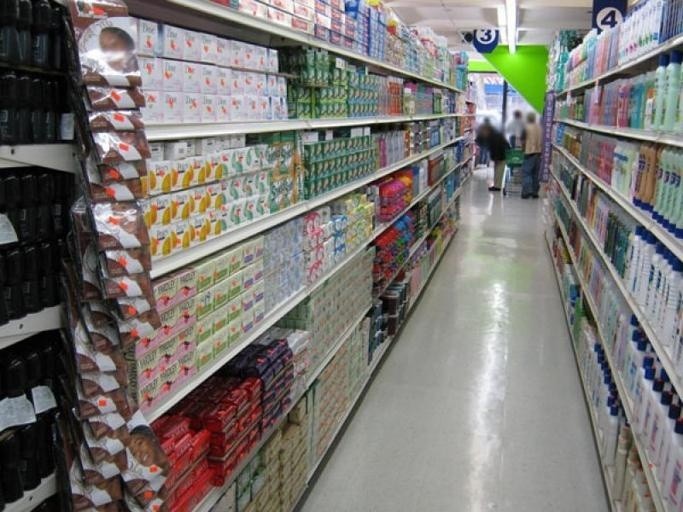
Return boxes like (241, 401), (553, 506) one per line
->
(475, 125), (510, 191)
(505, 110), (525, 148)
(519, 113), (543, 199)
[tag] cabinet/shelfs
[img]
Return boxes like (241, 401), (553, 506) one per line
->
(574, 0), (683, 512)
(541, 30), (573, 349)
(375, 1), (476, 366)
(0, 0), (375, 512)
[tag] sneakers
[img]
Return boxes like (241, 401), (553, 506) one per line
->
(521, 193), (539, 198)
(489, 186), (501, 192)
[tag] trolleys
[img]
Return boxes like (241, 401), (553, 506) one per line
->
(500, 147), (527, 198)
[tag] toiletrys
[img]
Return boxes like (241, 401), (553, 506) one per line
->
(582, 55), (683, 511)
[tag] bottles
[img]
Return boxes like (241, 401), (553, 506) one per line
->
(377, 128), (412, 300)
